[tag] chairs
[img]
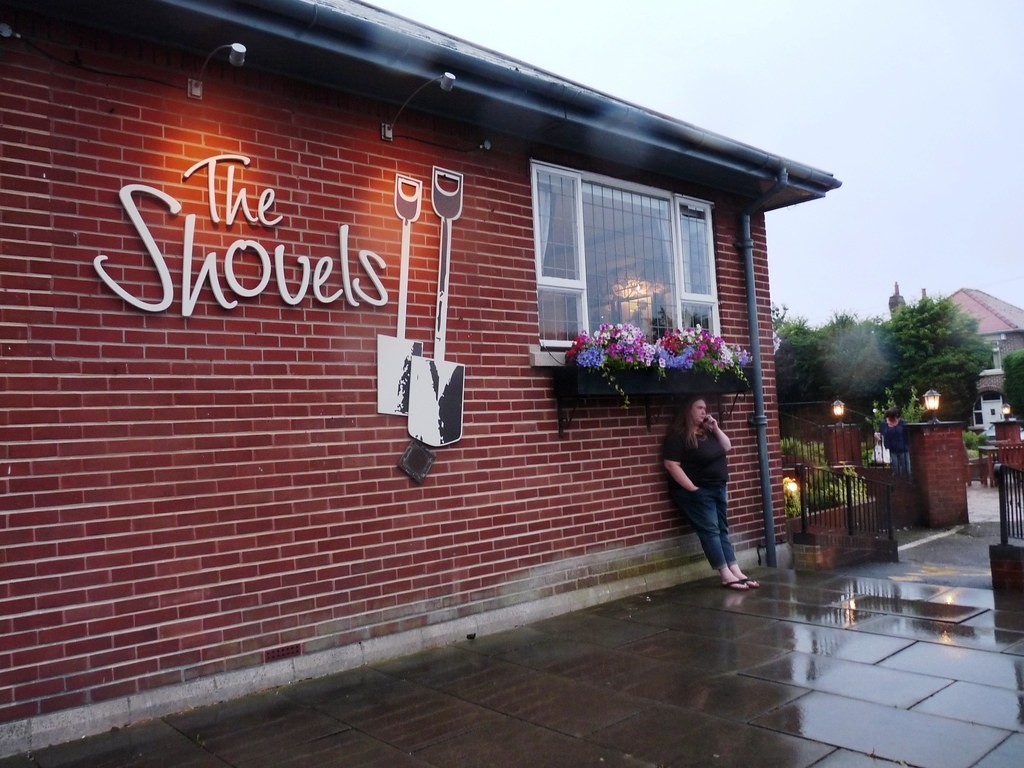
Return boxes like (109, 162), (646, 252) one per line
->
(994, 442), (1024, 468)
(962, 441), (987, 487)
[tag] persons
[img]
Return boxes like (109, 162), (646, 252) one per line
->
(663, 393), (760, 590)
(874, 406), (911, 478)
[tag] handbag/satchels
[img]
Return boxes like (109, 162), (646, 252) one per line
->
(871, 435), (892, 464)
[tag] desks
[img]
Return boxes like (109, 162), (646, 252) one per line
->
(977, 446), (1024, 488)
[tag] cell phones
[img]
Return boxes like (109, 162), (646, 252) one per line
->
(703, 418), (711, 424)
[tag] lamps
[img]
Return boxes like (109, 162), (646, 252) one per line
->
(831, 395), (847, 426)
(188, 42), (247, 100)
(380, 71), (456, 142)
(921, 387), (942, 423)
(999, 402), (1011, 422)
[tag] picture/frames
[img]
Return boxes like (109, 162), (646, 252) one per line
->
(618, 291), (654, 344)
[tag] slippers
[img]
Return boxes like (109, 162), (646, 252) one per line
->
(720, 576), (761, 591)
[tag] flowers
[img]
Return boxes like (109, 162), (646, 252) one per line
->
(553, 321), (755, 410)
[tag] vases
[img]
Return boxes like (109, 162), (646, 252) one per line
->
(553, 365), (755, 399)
